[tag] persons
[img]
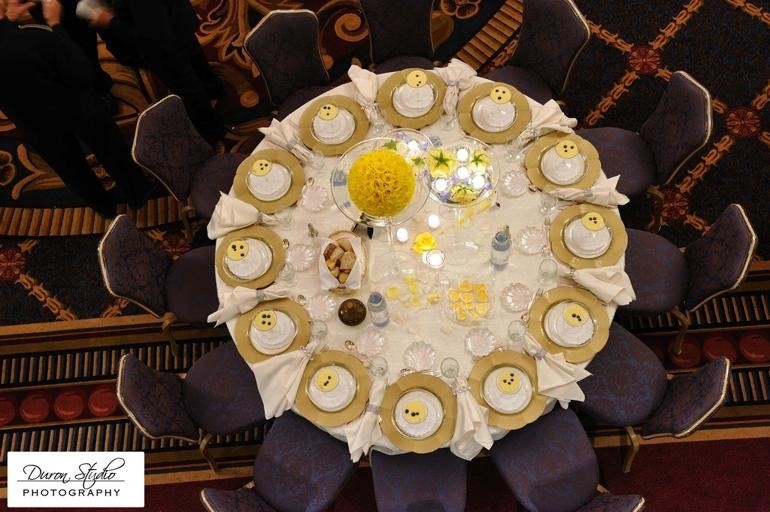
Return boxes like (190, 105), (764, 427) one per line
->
(0, 0), (224, 220)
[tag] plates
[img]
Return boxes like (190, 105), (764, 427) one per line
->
(216, 68), (628, 452)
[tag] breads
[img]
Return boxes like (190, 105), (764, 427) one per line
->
(323, 239), (357, 283)
